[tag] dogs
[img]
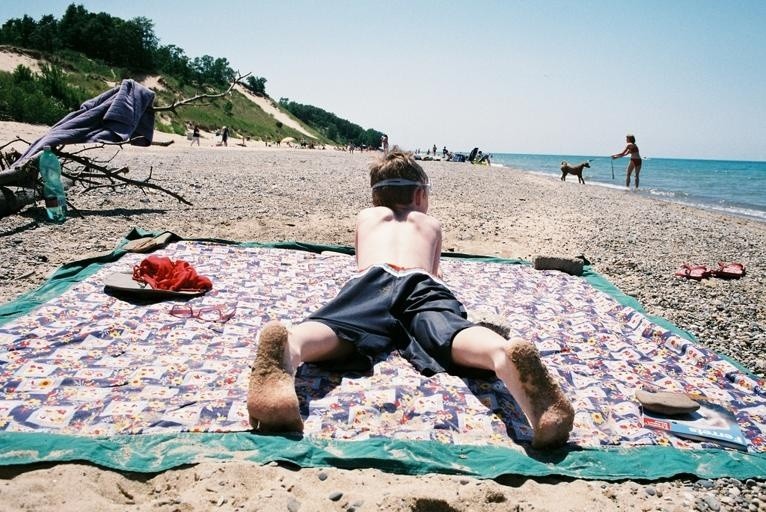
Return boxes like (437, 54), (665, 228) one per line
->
(560, 160), (591, 184)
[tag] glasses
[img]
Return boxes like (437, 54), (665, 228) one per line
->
(171, 303), (236, 321)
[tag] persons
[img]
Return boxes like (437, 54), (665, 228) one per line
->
(612, 134), (642, 188)
(248, 150), (573, 451)
(218, 125), (229, 146)
(190, 123), (200, 146)
(377, 136), (490, 165)
(275, 134), (373, 152)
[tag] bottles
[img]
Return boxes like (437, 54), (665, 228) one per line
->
(39, 146), (66, 222)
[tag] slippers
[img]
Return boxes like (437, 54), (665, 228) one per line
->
(713, 262), (746, 275)
(675, 263), (712, 277)
